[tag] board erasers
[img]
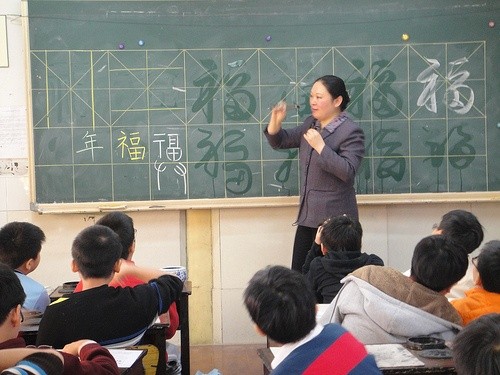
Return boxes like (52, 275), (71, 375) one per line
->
(98, 204), (129, 210)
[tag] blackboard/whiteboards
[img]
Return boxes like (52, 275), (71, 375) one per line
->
(20, 0), (500, 215)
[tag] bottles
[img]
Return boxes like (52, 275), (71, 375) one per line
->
(160, 266), (187, 285)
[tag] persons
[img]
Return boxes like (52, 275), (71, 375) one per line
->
(73, 211), (181, 375)
(244, 266), (383, 374)
(0, 263), (119, 375)
(452, 312), (500, 374)
(452, 239), (499, 327)
(0, 221), (51, 315)
(314, 233), (465, 346)
(34, 223), (185, 349)
(302, 213), (385, 304)
(261, 75), (364, 273)
(401, 208), (486, 302)
(1, 347), (65, 375)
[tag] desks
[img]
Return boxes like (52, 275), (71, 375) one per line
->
(105, 347), (148, 375)
(50, 282), (192, 375)
(256, 342), (457, 375)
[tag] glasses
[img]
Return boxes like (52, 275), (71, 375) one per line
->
(471, 255), (481, 272)
(18, 306), (24, 325)
(431, 223), (441, 231)
(132, 228), (137, 237)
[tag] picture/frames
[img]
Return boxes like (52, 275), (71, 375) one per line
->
(0, 15), (10, 67)
